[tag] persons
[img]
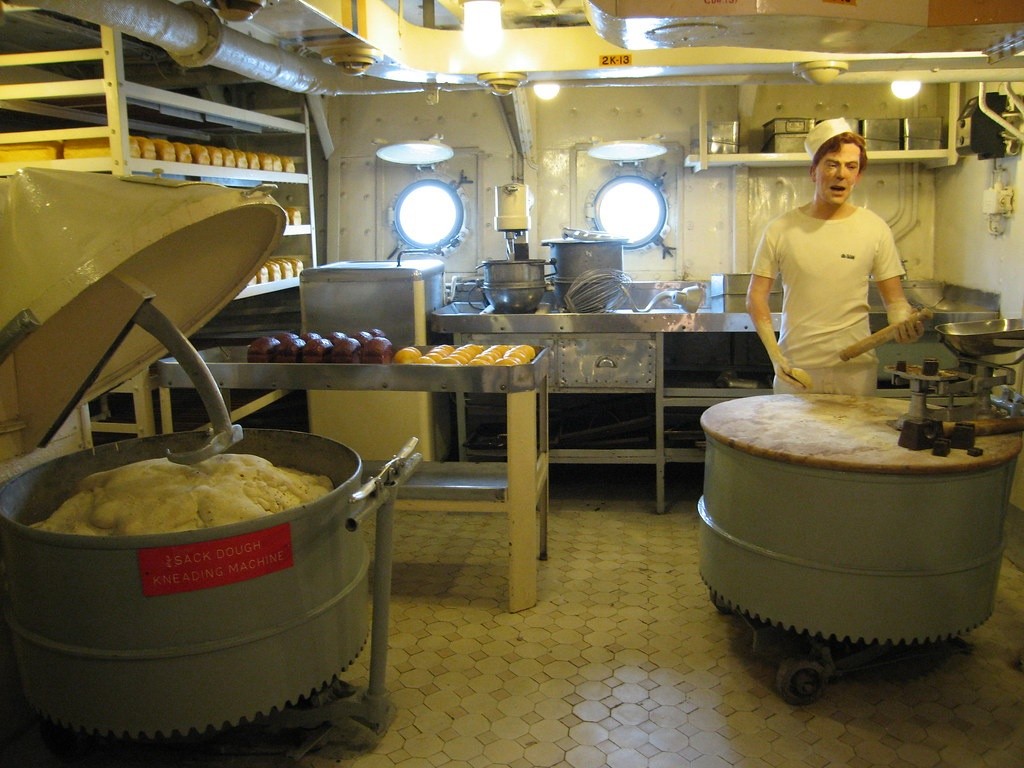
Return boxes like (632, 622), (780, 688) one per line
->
(745, 117), (925, 396)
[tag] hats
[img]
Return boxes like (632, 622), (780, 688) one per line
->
(804, 118), (852, 161)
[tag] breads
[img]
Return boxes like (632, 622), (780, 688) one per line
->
(241, 259), (304, 287)
(283, 207), (302, 225)
(392, 344), (536, 366)
(0, 136), (296, 173)
(245, 327), (393, 365)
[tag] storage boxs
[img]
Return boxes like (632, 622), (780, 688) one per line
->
(760, 116), (944, 153)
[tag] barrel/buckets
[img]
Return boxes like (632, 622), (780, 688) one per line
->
(553, 243), (625, 313)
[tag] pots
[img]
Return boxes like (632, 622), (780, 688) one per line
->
(473, 259), (557, 313)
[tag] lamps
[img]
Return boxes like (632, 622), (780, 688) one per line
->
(620, 283), (703, 314)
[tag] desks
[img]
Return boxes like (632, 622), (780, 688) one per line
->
(155, 342), (556, 616)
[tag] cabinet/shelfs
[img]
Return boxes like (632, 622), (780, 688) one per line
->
(0, 4), (318, 438)
